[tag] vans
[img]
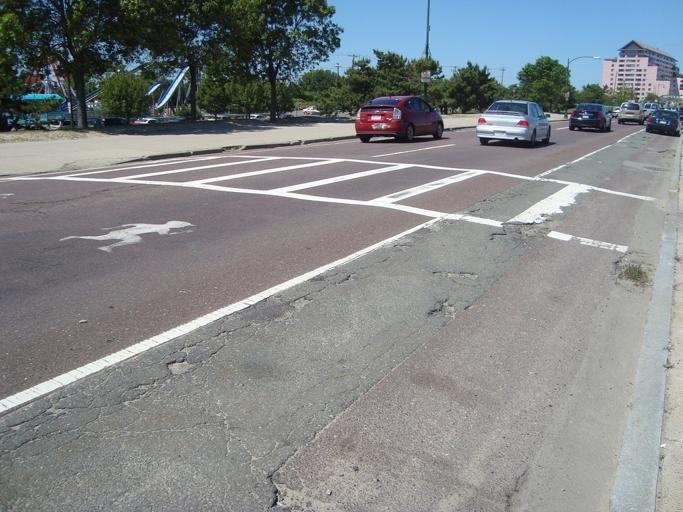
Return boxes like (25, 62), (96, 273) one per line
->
(2, 92), (68, 131)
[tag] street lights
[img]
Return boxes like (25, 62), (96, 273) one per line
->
(564, 55), (602, 118)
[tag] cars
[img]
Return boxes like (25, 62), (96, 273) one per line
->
(475, 98), (551, 147)
(568, 102), (613, 131)
(354, 94), (443, 143)
(612, 100), (683, 137)
